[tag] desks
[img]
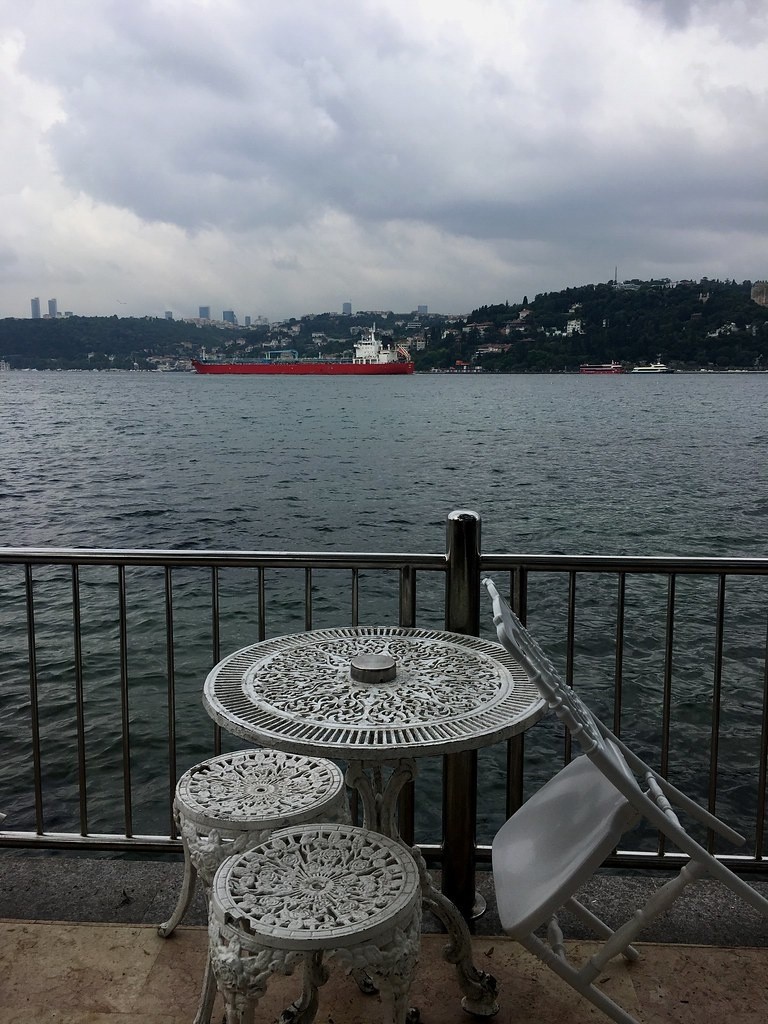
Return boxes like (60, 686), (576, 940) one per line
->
(200, 622), (549, 1024)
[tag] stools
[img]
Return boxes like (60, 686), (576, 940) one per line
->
(156, 747), (356, 939)
(191, 822), (423, 1024)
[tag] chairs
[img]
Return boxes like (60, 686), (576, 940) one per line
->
(481, 574), (768, 1024)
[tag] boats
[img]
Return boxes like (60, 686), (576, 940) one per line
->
(189, 318), (415, 376)
(577, 358), (626, 374)
(630, 358), (676, 375)
